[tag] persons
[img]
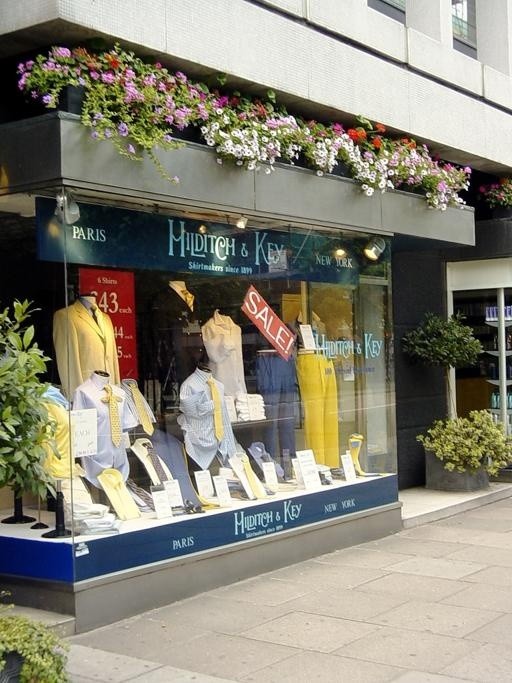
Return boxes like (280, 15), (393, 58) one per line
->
(71, 370), (140, 508)
(53, 296), (121, 402)
(179, 362), (234, 484)
(255, 350), (297, 480)
(201, 309), (245, 397)
(297, 347), (340, 471)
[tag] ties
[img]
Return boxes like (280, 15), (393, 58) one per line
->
(102, 384), (169, 511)
(181, 289), (194, 310)
(206, 379), (223, 442)
(242, 455), (291, 500)
(91, 304), (98, 336)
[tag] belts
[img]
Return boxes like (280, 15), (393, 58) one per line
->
(257, 352), (277, 356)
(298, 350), (326, 354)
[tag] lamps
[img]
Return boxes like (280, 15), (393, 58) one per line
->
(364, 235), (386, 260)
(50, 185), (82, 225)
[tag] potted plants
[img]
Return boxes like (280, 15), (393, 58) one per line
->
(414, 403), (510, 492)
